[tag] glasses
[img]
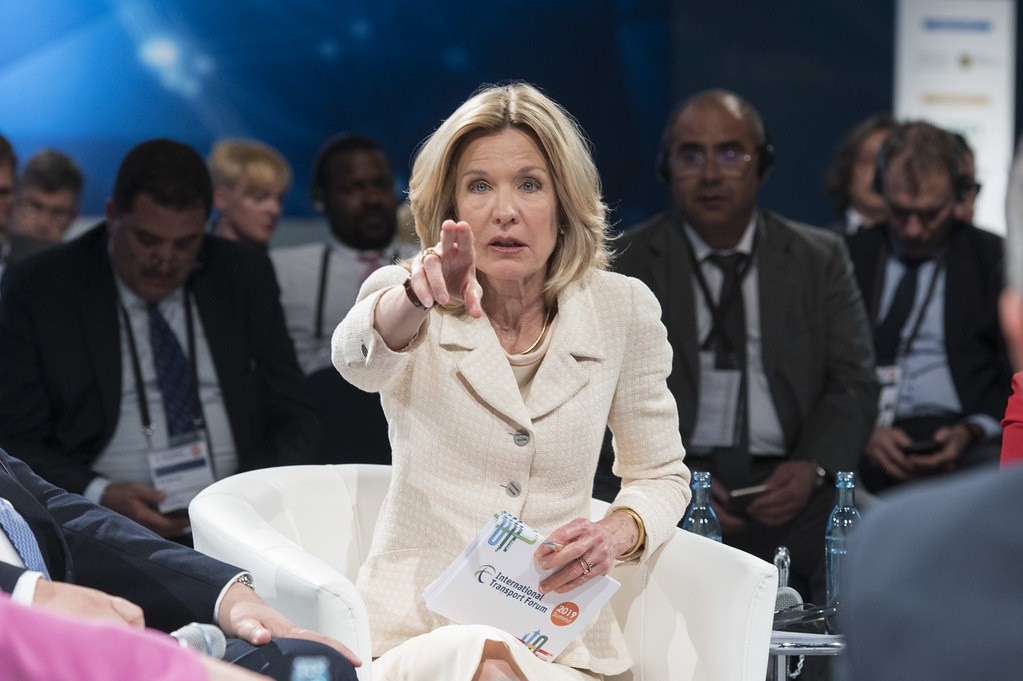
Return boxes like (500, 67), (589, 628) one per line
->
(670, 144), (765, 181)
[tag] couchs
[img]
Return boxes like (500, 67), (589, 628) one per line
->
(189, 464), (779, 681)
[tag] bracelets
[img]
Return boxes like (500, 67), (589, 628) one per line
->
(402, 278), (431, 314)
(612, 509), (644, 558)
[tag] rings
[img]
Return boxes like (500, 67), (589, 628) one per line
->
(579, 558), (592, 575)
(421, 247), (441, 263)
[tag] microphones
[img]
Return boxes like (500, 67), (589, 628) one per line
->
(170, 622), (225, 661)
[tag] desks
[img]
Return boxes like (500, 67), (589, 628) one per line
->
(765, 642), (846, 681)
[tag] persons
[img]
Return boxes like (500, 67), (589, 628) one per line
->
(589, 91), (880, 681)
(270, 132), (422, 465)
(819, 118), (1022, 498)
(327, 82), (694, 681)
(0, 135), (356, 681)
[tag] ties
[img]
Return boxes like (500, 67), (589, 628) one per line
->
(0, 496), (52, 583)
(877, 256), (925, 367)
(145, 301), (207, 445)
(706, 253), (752, 444)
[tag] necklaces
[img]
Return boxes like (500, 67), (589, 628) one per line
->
(488, 299), (552, 354)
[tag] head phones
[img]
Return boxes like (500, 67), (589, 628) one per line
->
(659, 142), (776, 184)
(872, 166), (972, 203)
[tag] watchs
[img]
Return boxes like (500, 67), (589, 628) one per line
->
(236, 574), (256, 589)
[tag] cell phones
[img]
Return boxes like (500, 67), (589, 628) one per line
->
(903, 439), (938, 456)
(165, 507), (190, 518)
(729, 484), (770, 505)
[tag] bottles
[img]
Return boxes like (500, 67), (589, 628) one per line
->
(682, 471), (722, 544)
(291, 656), (331, 681)
(824, 470), (862, 608)
